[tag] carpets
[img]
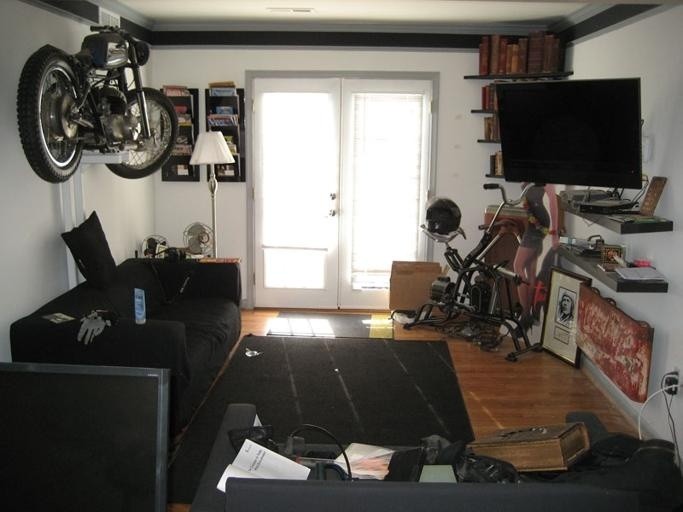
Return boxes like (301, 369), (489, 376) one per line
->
(163, 332), (477, 503)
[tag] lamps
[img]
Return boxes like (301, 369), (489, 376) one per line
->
(190, 130), (235, 257)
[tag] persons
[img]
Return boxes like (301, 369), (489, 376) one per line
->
(511, 183), (561, 337)
(559, 291), (574, 321)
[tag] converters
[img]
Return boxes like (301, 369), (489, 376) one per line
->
(665, 377), (678, 394)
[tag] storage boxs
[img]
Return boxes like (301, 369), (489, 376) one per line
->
(387, 261), (450, 313)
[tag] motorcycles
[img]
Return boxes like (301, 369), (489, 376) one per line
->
(14, 22), (180, 184)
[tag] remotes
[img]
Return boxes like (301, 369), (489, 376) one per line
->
(608, 215), (634, 224)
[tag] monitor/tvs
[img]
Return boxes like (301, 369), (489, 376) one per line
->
(495, 76), (641, 188)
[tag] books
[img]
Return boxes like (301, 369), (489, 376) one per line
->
(158, 81), (240, 156)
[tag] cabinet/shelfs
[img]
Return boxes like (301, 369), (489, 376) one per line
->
(555, 192), (673, 294)
(462, 71), (576, 179)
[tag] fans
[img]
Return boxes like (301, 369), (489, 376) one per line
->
(182, 221), (214, 257)
(141, 234), (167, 259)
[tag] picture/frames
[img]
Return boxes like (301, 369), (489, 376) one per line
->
(537, 266), (592, 369)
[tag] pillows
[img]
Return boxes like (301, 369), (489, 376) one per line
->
(60, 211), (115, 281)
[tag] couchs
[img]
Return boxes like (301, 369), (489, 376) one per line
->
(190, 403), (682, 511)
(10, 257), (242, 447)
